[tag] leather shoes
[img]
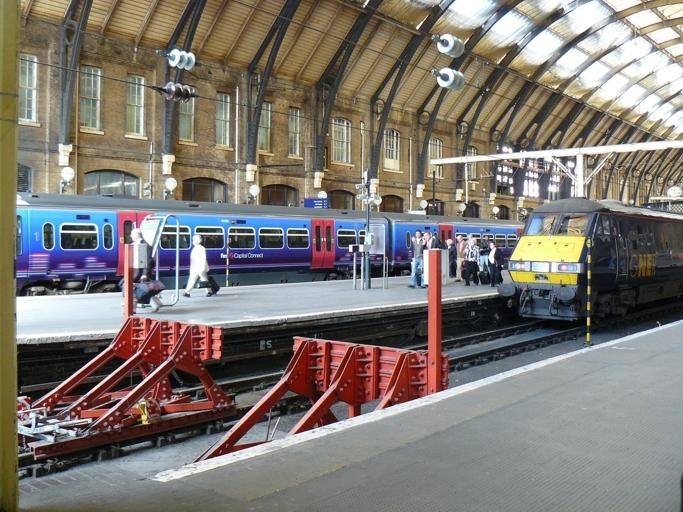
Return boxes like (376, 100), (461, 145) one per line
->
(207, 292), (212, 296)
(182, 293), (190, 297)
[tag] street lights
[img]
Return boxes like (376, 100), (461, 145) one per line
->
(355, 178), (382, 289)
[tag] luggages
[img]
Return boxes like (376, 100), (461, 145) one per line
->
(206, 277), (220, 292)
(479, 271), (489, 285)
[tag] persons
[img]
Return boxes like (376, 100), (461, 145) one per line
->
(122, 229), (159, 314)
(406, 228), (503, 288)
(181, 234), (214, 297)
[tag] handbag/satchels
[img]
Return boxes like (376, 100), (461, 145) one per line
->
(134, 280), (166, 304)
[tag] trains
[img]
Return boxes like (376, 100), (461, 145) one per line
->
(16, 192), (527, 297)
(508, 199), (683, 323)
